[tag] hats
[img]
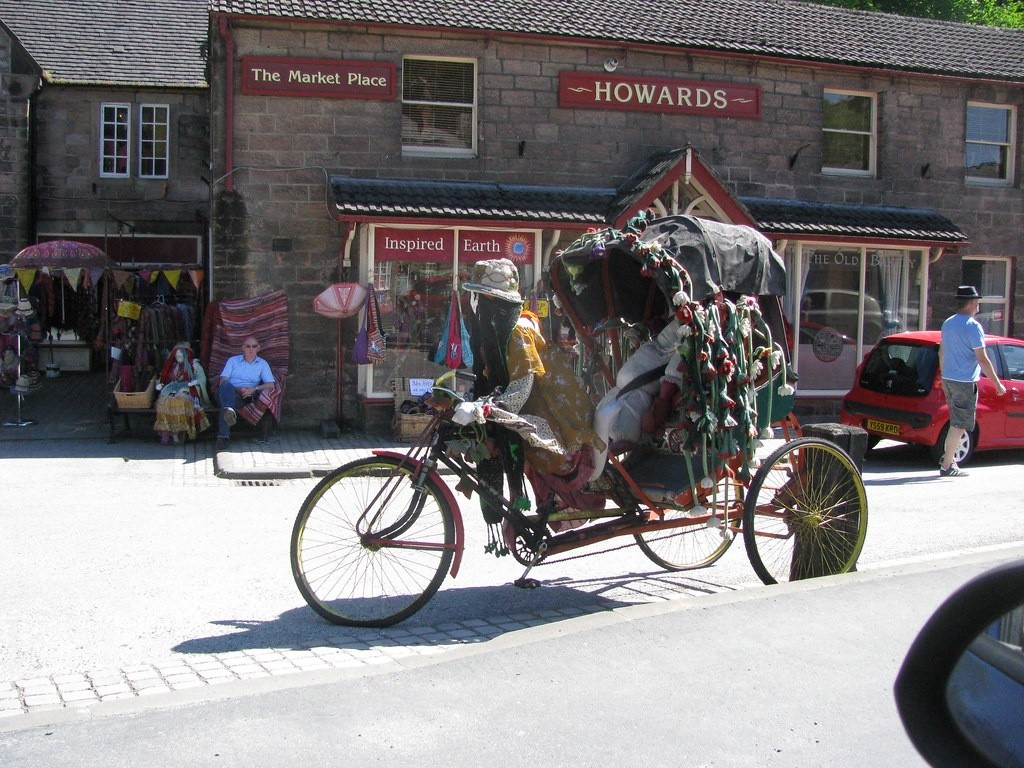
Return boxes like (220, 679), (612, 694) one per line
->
(173, 342), (194, 353)
(461, 258), (524, 305)
(954, 286), (982, 298)
(0, 298), (43, 394)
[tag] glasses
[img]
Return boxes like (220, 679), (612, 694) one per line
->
(245, 344), (257, 348)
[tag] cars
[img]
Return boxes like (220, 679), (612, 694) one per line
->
(839, 331), (1024, 468)
(798, 288), (885, 345)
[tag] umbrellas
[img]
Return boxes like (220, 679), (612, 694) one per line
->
(7, 240), (114, 323)
(312, 281), (367, 318)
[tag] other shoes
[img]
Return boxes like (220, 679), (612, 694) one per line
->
(224, 407), (237, 426)
(219, 437), (229, 450)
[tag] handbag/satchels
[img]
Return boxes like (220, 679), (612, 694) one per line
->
(367, 290), (386, 359)
(428, 290), (473, 369)
(352, 283), (374, 365)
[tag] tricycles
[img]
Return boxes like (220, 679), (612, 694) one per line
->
(289, 211), (871, 629)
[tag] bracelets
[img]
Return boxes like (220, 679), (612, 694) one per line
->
(255, 386), (258, 392)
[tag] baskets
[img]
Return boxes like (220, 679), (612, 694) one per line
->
(112, 371), (158, 409)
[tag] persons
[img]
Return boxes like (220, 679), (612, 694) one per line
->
(450, 258), (606, 533)
(938, 286), (1006, 476)
(214, 337), (275, 450)
(153, 342), (212, 445)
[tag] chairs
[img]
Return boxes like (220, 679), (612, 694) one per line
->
(197, 289), (289, 443)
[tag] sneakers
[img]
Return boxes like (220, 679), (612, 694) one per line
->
(939, 455), (961, 470)
(940, 462), (968, 477)
